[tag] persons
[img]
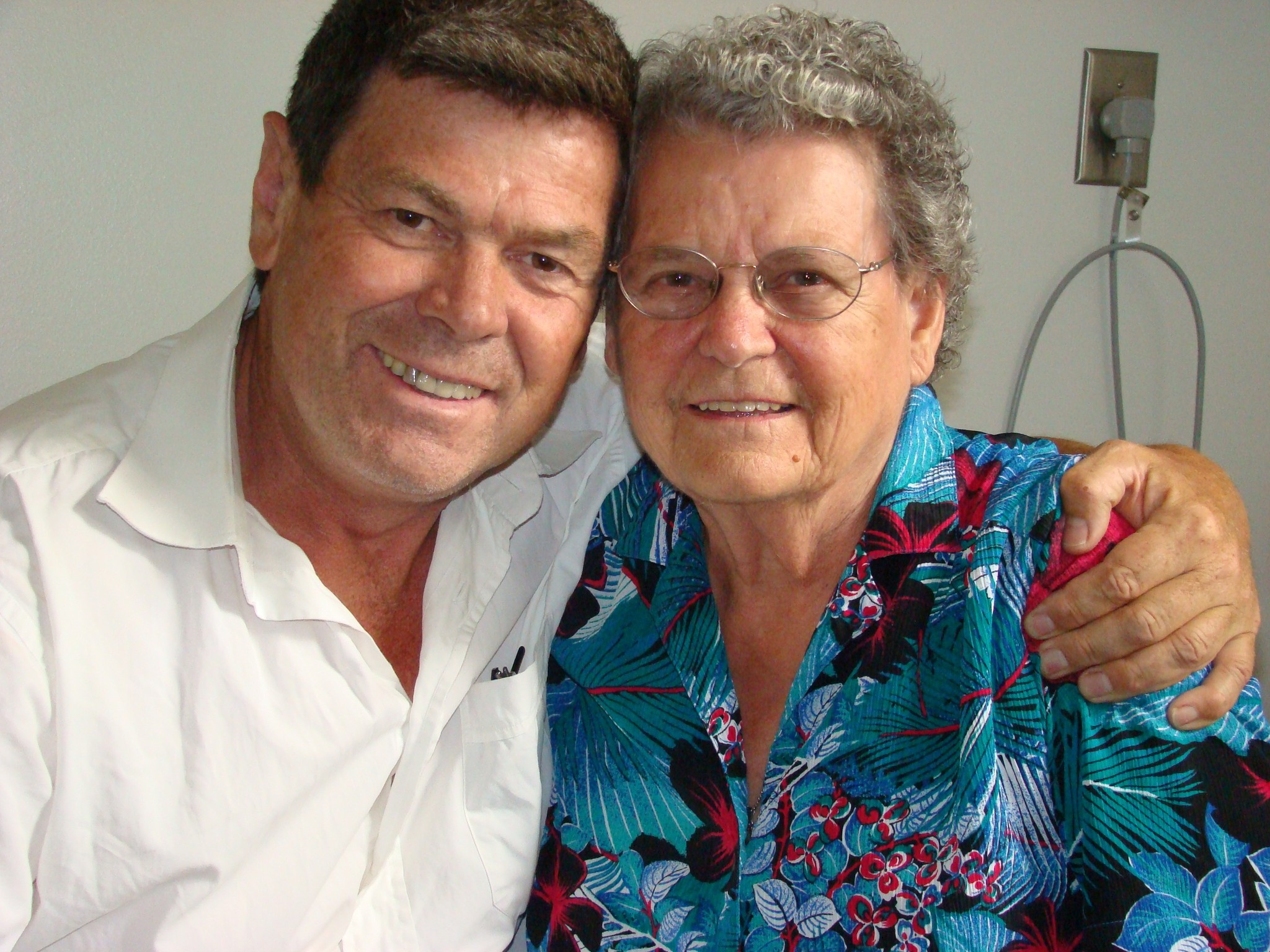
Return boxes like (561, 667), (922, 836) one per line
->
(506, 9), (1270, 952)
(1, 1), (1262, 952)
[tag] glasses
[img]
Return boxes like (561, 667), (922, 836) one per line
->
(606, 246), (897, 319)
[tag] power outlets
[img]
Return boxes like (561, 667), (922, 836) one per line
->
(1074, 47), (1159, 190)
